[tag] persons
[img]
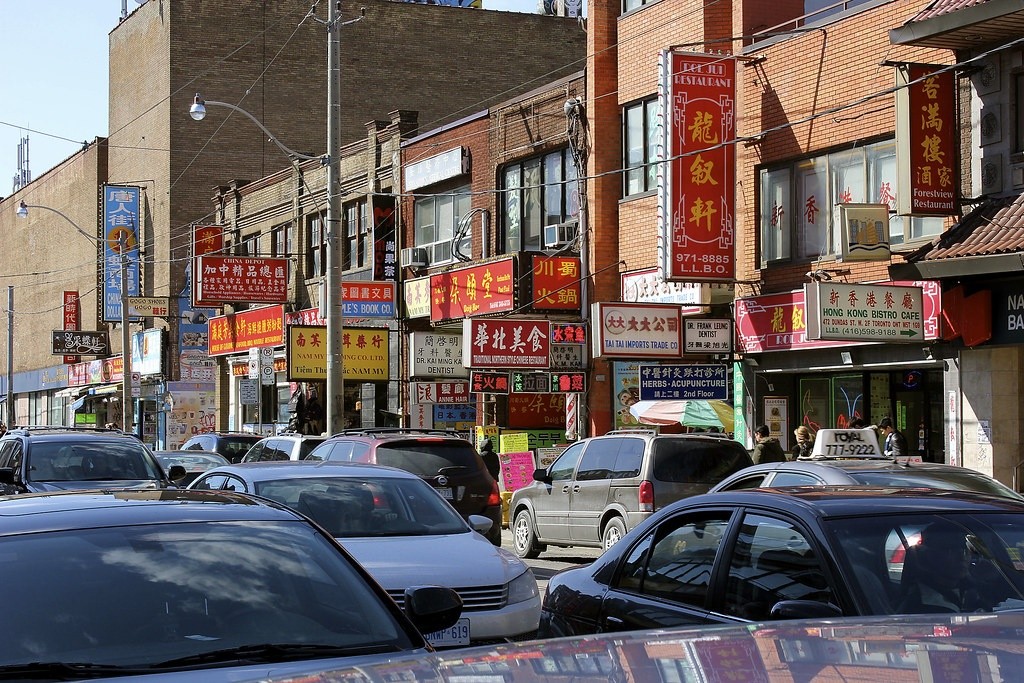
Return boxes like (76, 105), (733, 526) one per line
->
(692, 427), (734, 439)
(752, 425), (787, 466)
(480, 439), (501, 482)
(0, 424), (6, 436)
(106, 422), (121, 430)
(791, 416), (910, 460)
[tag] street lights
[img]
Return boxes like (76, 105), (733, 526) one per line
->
(188, 1), (345, 435)
(16, 199), (133, 435)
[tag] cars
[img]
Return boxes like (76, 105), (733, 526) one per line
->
(537, 484), (1024, 641)
(152, 450), (232, 489)
(0, 489), (437, 683)
(185, 460), (543, 653)
(666, 458), (1024, 583)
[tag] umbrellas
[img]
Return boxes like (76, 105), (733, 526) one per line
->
(630, 399), (733, 434)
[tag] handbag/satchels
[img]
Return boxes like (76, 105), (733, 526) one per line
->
(286, 385), (302, 412)
(306, 380), (318, 401)
(281, 397), (324, 436)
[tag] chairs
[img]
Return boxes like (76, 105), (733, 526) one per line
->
(325, 486), (375, 512)
(901, 545), (928, 596)
(754, 550), (817, 598)
(909, 577), (911, 578)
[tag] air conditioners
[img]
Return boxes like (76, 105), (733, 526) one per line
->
(544, 224), (575, 247)
(401, 248), (426, 267)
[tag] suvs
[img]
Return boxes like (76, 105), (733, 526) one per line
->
(304, 426), (503, 550)
(506, 428), (755, 560)
(240, 434), (327, 460)
(0, 425), (179, 497)
(178, 435), (262, 465)
